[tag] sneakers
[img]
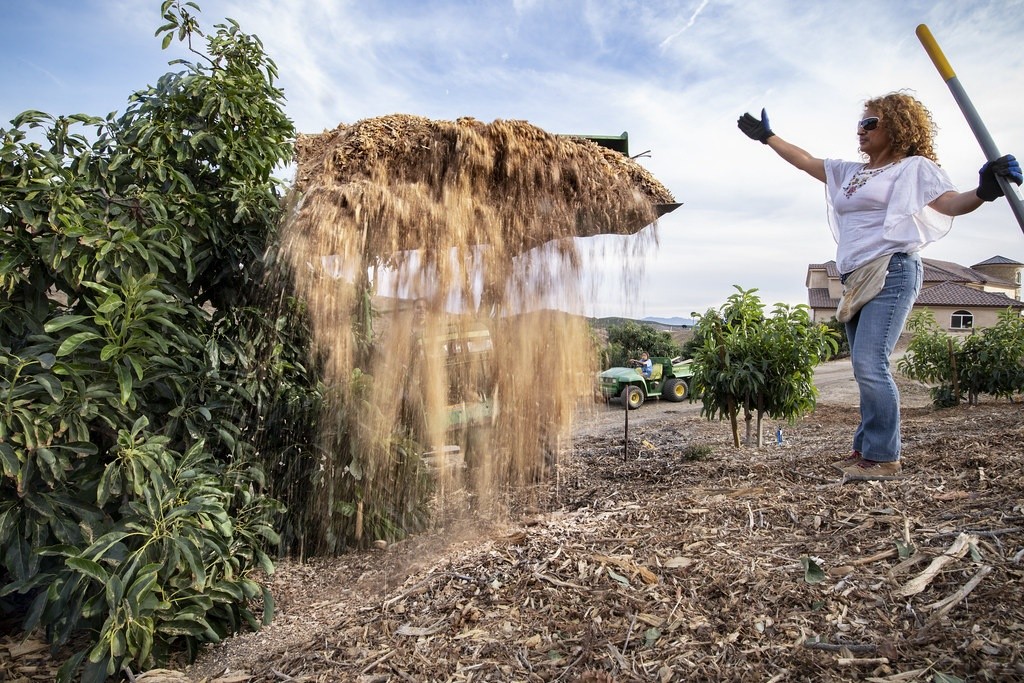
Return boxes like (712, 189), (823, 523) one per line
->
(831, 451), (861, 471)
(843, 458), (904, 480)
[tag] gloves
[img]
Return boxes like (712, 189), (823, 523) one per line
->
(737, 108), (775, 145)
(976, 154), (1023, 202)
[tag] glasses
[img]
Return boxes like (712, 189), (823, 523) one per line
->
(858, 117), (880, 132)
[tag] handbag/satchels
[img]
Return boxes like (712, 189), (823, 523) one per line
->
(835, 254), (893, 324)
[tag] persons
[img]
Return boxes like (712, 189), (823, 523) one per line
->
(738, 94), (1023, 478)
(630, 352), (652, 378)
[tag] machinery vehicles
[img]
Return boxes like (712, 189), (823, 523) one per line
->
(297, 131), (684, 470)
(598, 357), (706, 410)
